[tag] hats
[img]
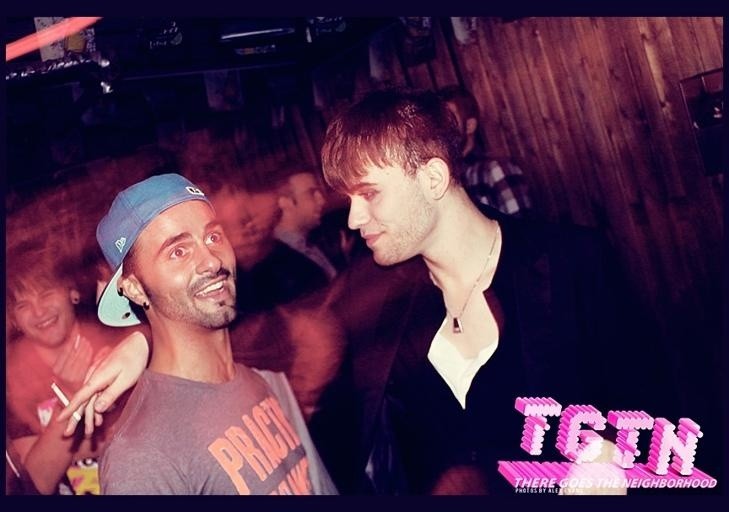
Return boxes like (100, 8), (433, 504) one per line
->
(96, 174), (217, 328)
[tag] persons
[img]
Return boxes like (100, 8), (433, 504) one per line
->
(6, 85), (728, 497)
